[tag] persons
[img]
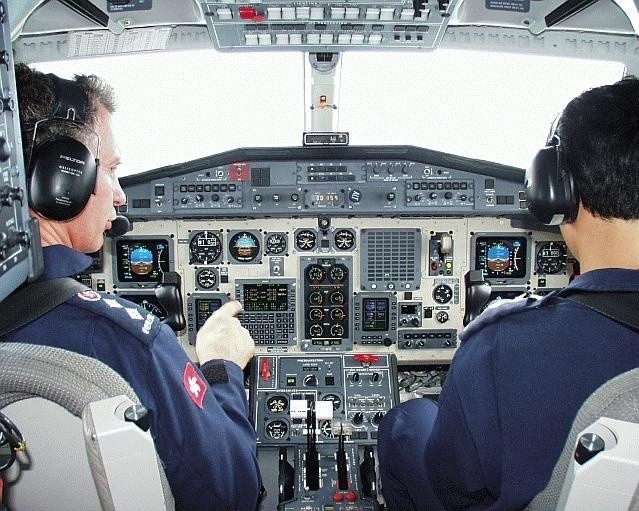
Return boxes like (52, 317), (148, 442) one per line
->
(1, 58), (266, 511)
(375, 74), (639, 511)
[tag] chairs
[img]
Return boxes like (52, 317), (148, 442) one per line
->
(0, 342), (175, 511)
(524, 367), (639, 511)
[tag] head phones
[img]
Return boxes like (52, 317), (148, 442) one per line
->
(26, 72), (100, 223)
(523, 110), (580, 226)
